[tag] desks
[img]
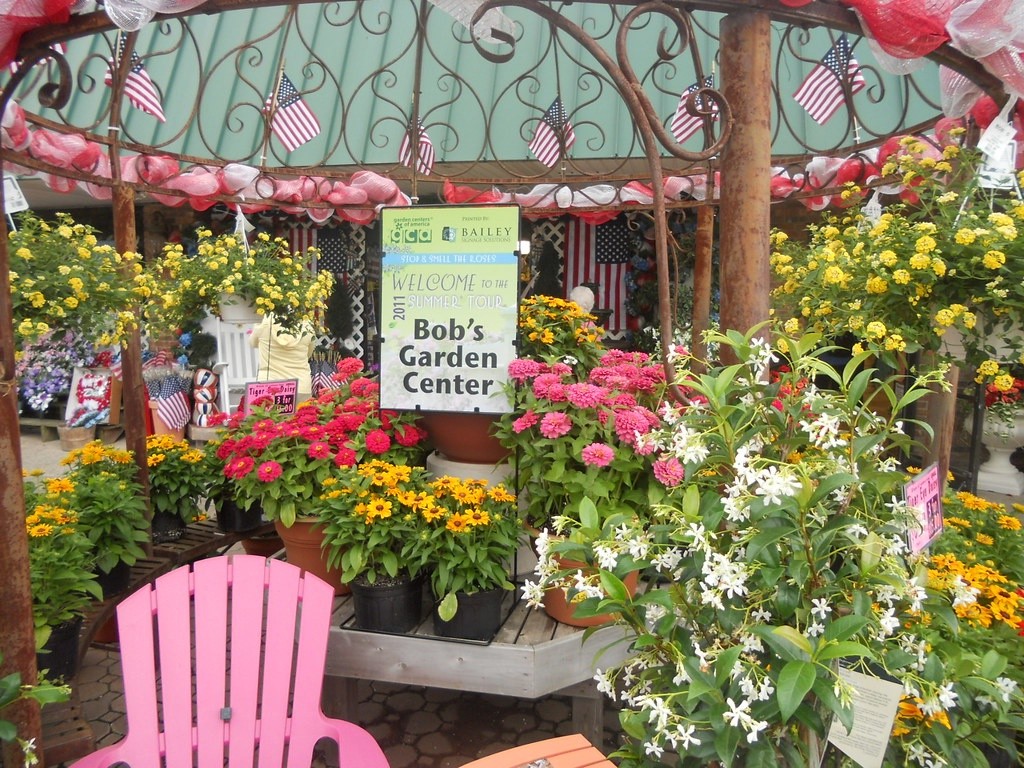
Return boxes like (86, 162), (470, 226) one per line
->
(590, 309), (614, 327)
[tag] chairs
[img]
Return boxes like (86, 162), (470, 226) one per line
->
(211, 316), (274, 417)
(67, 555), (390, 768)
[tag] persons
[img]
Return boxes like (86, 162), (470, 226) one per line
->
(535, 240), (562, 298)
(248, 308), (312, 406)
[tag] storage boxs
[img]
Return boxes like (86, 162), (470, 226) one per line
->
(65, 367), (122, 425)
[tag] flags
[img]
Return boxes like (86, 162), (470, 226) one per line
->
(527, 96), (575, 170)
(260, 70), (321, 153)
(8, 41), (67, 78)
(563, 217), (628, 331)
(791, 32), (865, 127)
(144, 371), (192, 432)
(104, 29), (168, 124)
(669, 75), (719, 144)
(398, 91), (435, 176)
(309, 360), (349, 399)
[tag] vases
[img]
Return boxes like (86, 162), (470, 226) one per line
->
(147, 400), (185, 443)
(213, 494), (262, 532)
(810, 330), (866, 408)
(57, 425), (96, 452)
(416, 410), (511, 464)
(926, 300), (1024, 365)
(431, 585), (503, 640)
(347, 566), (424, 633)
(522, 514), (656, 628)
(36, 608), (83, 687)
(218, 290), (264, 324)
(152, 498), (188, 545)
(274, 515), (352, 596)
(85, 547), (131, 602)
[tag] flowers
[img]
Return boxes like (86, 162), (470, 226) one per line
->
(6, 126), (1024, 768)
